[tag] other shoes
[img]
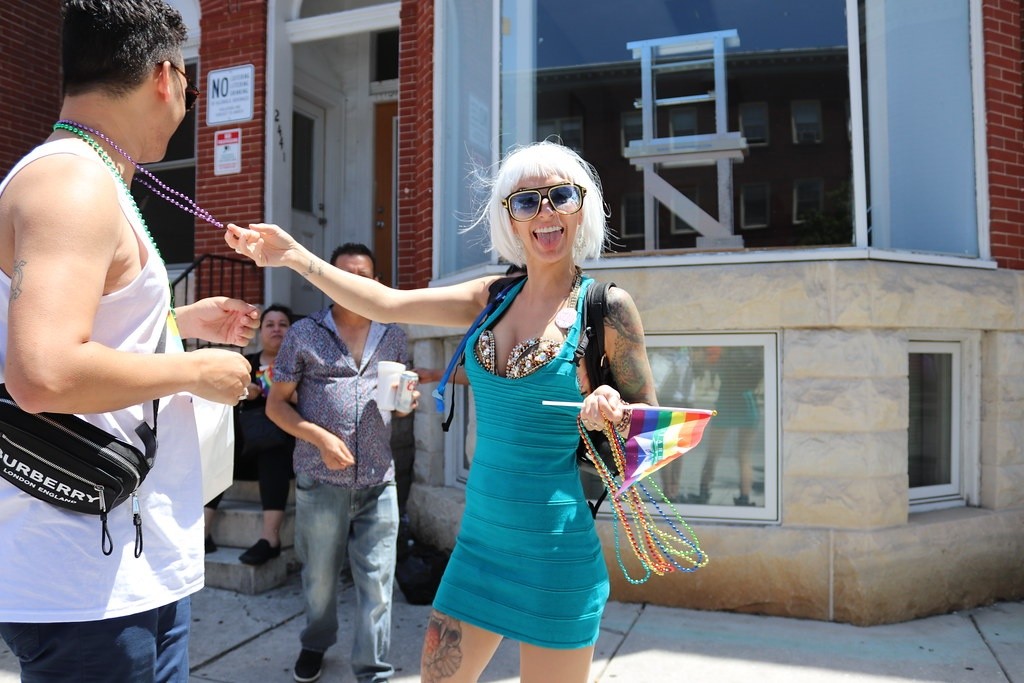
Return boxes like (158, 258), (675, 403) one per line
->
(240, 537), (282, 564)
(734, 496), (754, 506)
(667, 494), (686, 502)
(292, 648), (322, 681)
(687, 491), (710, 503)
(205, 533), (216, 554)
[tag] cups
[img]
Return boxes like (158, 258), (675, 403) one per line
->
(376, 360), (407, 411)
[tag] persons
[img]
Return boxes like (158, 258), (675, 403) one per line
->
(0, 1), (264, 680)
(204, 302), (297, 567)
(222, 139), (666, 681)
(692, 347), (720, 389)
(660, 454), (682, 504)
(687, 346), (761, 506)
(264, 242), (420, 683)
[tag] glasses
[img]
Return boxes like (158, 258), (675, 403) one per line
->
(158, 60), (201, 111)
(502, 182), (586, 223)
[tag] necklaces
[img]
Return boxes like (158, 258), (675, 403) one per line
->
(53, 124), (176, 318)
(56, 120), (223, 229)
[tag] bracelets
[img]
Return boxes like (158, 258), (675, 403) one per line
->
(615, 398), (631, 434)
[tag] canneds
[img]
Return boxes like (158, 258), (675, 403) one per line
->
(393, 371), (419, 414)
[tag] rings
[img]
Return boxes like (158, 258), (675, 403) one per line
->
(235, 249), (241, 254)
(239, 387), (250, 400)
(589, 422), (598, 429)
(580, 418), (588, 424)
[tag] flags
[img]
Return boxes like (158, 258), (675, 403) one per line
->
(613, 409), (712, 499)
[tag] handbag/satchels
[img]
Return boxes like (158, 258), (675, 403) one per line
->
(1, 385), (150, 515)
(397, 532), (452, 608)
